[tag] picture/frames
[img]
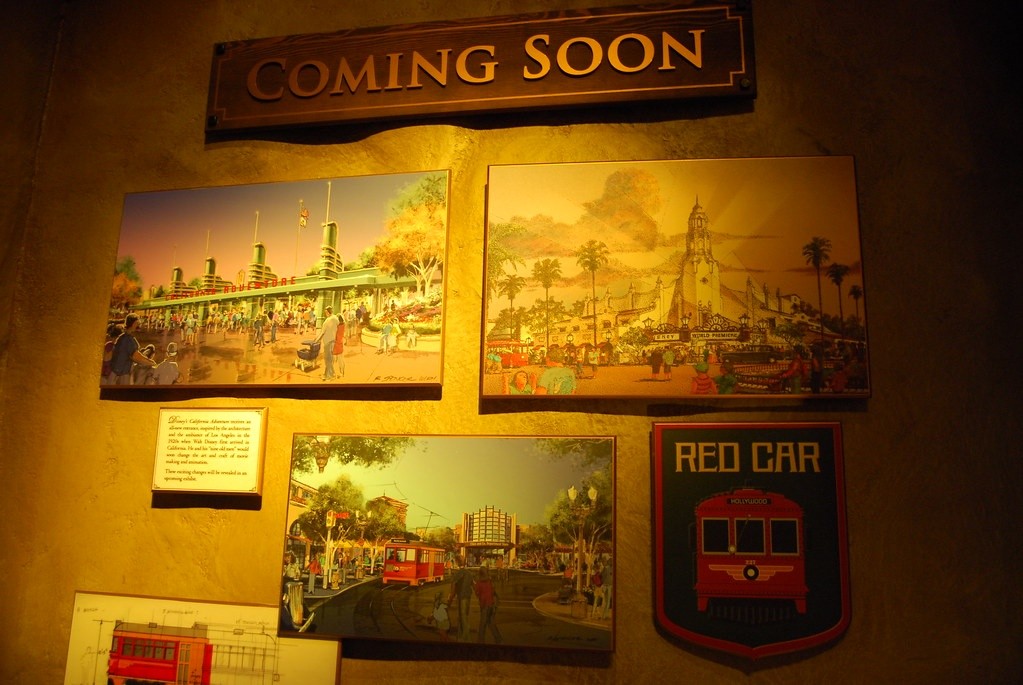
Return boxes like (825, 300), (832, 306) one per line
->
(99, 168), (451, 396)
(477, 155), (874, 408)
(650, 422), (852, 665)
(277, 431), (620, 657)
